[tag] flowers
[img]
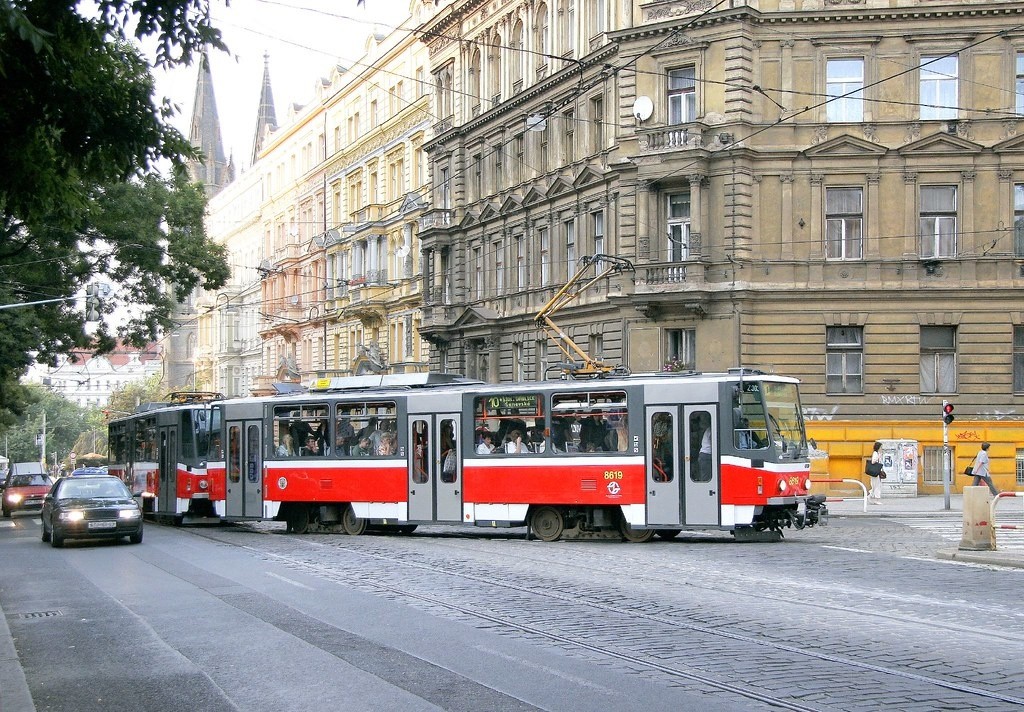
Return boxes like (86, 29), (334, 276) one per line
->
(663, 356), (686, 372)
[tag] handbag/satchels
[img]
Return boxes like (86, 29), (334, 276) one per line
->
(443, 449), (456, 474)
(964, 456), (977, 476)
(865, 458), (883, 477)
(879, 471), (886, 479)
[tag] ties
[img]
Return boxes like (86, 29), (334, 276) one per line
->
(745, 434), (748, 444)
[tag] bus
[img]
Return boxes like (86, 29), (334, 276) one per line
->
(107, 391), (227, 524)
(205, 367), (824, 544)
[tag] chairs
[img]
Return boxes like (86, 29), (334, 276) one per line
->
(65, 486), (82, 497)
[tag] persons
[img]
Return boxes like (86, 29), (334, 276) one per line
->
(970, 441), (998, 498)
(866, 441), (885, 507)
(264, 406), (760, 490)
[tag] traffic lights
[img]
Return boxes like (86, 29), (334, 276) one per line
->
(105, 409), (109, 419)
(943, 403), (955, 424)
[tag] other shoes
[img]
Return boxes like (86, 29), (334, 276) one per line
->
(867, 496), (882, 505)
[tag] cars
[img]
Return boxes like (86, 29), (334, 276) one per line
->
(0, 471), (6, 489)
(2, 473), (55, 517)
(69, 466), (109, 477)
(40, 475), (144, 548)
